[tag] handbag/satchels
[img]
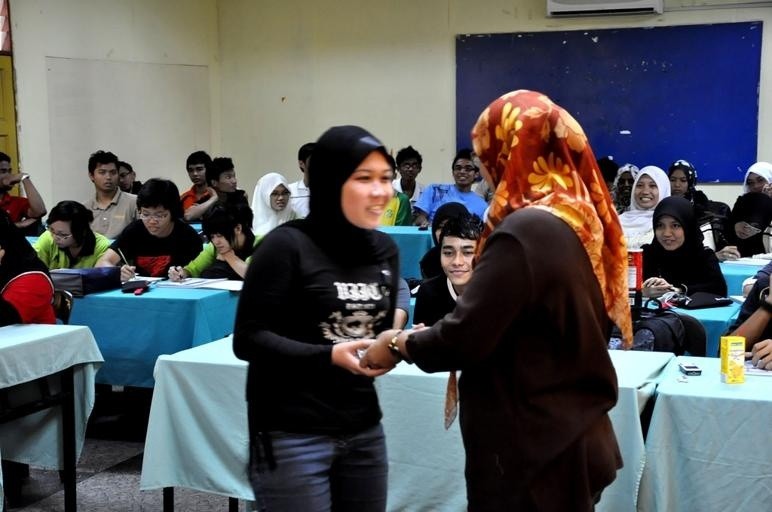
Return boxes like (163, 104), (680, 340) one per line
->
(50, 267), (120, 295)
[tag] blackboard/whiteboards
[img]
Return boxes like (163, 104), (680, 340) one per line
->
(44, 56), (213, 224)
(452, 19), (765, 187)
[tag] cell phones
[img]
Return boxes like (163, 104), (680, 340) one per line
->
(419, 224), (428, 230)
(678, 362), (701, 376)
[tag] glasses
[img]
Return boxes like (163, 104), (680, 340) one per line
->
(139, 211), (168, 219)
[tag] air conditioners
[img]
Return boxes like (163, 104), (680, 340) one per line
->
(546, 0), (665, 19)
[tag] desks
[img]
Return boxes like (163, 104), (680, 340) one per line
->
(638, 292), (747, 357)
(140, 332), (675, 512)
(25, 223), (242, 392)
(719, 261), (769, 297)
(636, 355), (771, 512)
(380, 223), (436, 284)
(0, 321), (105, 512)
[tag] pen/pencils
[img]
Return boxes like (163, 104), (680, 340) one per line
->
(116, 246), (136, 279)
(174, 265), (183, 280)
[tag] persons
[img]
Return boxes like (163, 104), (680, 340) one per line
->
(598, 155), (772, 371)
(233, 125), (397, 511)
(0, 142), (316, 325)
(382, 144), (493, 329)
(360, 90), (633, 509)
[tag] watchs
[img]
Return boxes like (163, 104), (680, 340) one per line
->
(387, 327), (404, 364)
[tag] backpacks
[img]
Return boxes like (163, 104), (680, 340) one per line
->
(607, 298), (687, 355)
